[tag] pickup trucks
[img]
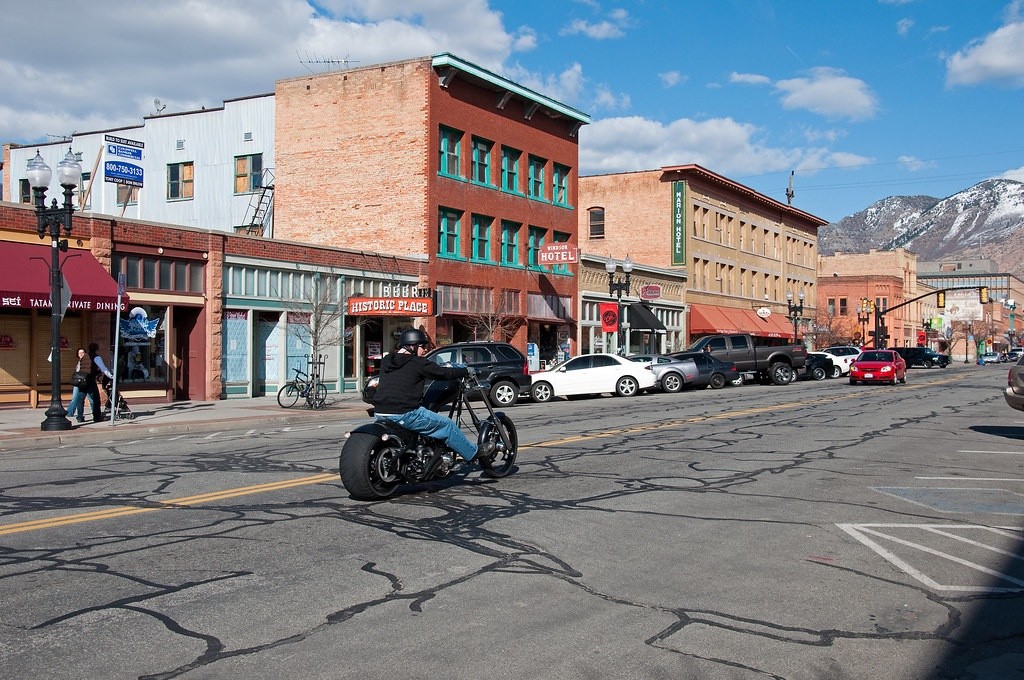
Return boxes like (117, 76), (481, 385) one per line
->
(690, 333), (808, 385)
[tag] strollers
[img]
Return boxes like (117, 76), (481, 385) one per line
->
(98, 371), (138, 424)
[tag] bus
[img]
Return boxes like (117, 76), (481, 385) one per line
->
(1010, 346), (1024, 352)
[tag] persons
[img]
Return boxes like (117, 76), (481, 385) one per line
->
(373, 328), (495, 462)
(66, 343), (113, 423)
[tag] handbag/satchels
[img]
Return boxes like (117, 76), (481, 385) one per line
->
(70, 372), (90, 387)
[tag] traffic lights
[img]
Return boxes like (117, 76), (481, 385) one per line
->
(862, 300), (875, 313)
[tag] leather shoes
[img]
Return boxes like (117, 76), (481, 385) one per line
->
(468, 441), (495, 462)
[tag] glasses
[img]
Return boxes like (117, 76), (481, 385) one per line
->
(418, 344), (425, 347)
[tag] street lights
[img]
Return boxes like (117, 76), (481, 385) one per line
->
(25, 143), (85, 434)
(937, 291), (945, 308)
(785, 286), (808, 346)
(604, 253), (635, 358)
(961, 317), (972, 364)
(979, 286), (989, 304)
(856, 304), (875, 346)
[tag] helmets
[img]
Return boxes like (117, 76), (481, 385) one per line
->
(395, 330), (429, 346)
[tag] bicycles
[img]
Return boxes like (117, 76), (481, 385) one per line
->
(276, 369), (327, 409)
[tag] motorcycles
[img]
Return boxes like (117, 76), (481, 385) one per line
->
(339, 368), (518, 502)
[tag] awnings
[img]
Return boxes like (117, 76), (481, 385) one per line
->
(0, 240), (130, 312)
(630, 303), (667, 333)
(691, 304), (802, 339)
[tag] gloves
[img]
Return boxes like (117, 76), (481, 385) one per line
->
(467, 366), (475, 377)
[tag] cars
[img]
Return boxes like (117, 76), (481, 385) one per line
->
(531, 353), (699, 398)
(850, 350), (907, 385)
(667, 352), (740, 388)
(806, 352), (850, 381)
(981, 352), (1024, 364)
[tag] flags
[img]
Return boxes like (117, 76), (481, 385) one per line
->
(918, 332), (926, 345)
(600, 303), (619, 332)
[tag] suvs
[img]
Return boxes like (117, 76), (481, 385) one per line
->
(887, 346), (950, 369)
(820, 346), (867, 376)
(1003, 354), (1024, 413)
(362, 342), (533, 412)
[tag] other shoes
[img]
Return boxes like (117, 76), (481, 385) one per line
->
(77, 419), (85, 422)
(94, 415), (103, 422)
